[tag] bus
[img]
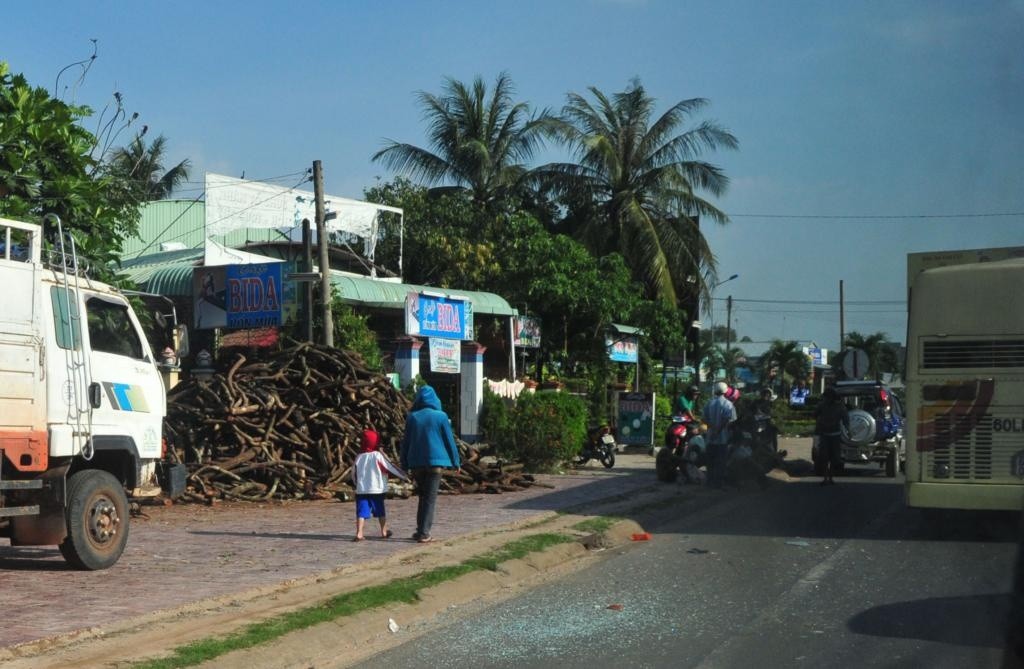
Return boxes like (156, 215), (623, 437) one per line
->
(905, 247), (1024, 520)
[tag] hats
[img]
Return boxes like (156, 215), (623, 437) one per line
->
(715, 382), (727, 394)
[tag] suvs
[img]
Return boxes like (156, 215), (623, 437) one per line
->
(811, 380), (906, 478)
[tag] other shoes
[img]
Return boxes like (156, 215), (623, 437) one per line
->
(413, 532), (436, 543)
(354, 536), (365, 541)
(384, 529), (392, 539)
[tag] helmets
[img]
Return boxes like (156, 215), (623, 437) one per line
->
(689, 385), (701, 394)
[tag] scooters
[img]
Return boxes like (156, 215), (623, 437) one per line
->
(728, 414), (779, 485)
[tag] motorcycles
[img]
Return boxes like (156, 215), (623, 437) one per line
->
(571, 425), (618, 468)
(655, 414), (705, 483)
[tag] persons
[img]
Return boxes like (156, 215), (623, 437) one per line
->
(397, 384), (461, 543)
(668, 381), (774, 491)
(351, 429), (409, 541)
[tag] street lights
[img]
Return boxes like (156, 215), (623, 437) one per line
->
(710, 274), (739, 402)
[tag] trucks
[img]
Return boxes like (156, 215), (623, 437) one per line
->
(0, 211), (190, 569)
